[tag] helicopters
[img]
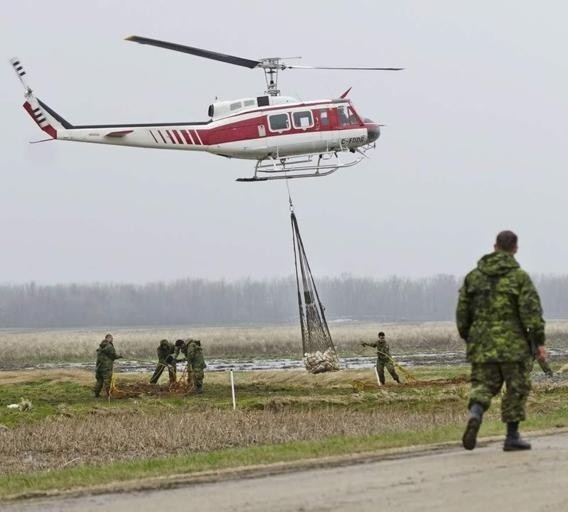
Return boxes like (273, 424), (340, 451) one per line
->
(9, 35), (404, 182)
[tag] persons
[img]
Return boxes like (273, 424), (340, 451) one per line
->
(362, 332), (403, 386)
(95, 333), (124, 397)
(170, 338), (207, 394)
(456, 230), (546, 451)
(149, 339), (179, 384)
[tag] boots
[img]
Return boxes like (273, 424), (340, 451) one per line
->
(504, 422), (531, 451)
(463, 403), (485, 449)
